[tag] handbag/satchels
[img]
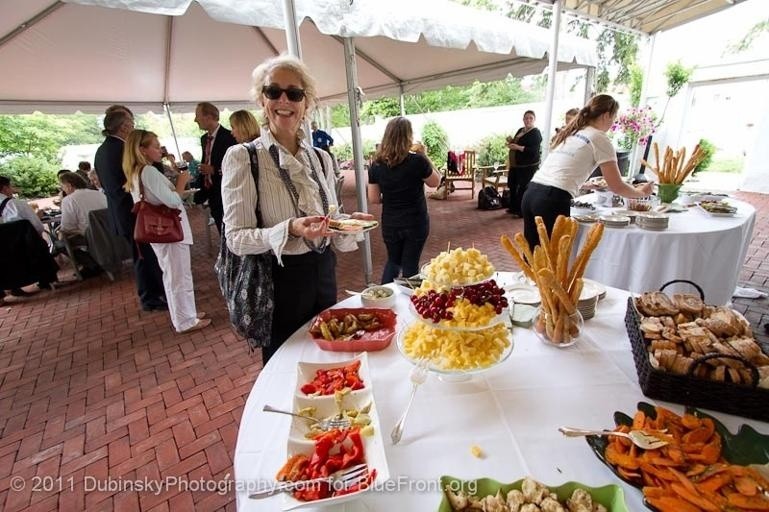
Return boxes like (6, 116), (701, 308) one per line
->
(478, 187), (503, 210)
(131, 168), (183, 243)
(214, 222), (275, 349)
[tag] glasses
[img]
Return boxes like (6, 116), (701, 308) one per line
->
(263, 85), (307, 101)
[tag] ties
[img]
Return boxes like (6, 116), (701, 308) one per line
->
(204, 136), (213, 189)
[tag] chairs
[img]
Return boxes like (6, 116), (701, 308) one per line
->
(442, 149), (476, 200)
(0, 209), (134, 290)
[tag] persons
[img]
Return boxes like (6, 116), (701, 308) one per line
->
(556, 109), (581, 130)
(521, 96), (653, 275)
(368, 114), (442, 285)
(0, 103), (261, 334)
(309, 120), (334, 151)
(506, 109), (544, 220)
(219, 52), (373, 370)
(321, 144), (342, 178)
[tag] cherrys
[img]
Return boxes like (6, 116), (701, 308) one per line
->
(408, 280), (509, 323)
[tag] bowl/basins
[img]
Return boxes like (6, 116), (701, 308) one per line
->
(360, 286), (395, 308)
(680, 194), (698, 204)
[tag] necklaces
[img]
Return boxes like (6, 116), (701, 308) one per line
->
(264, 138), (334, 255)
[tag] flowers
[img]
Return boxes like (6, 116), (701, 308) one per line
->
(611, 104), (662, 149)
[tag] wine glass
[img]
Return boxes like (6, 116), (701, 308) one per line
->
(53, 198), (62, 209)
(178, 160), (188, 173)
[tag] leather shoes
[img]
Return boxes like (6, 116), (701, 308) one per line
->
(197, 312), (206, 318)
(143, 297), (168, 311)
(179, 318), (212, 334)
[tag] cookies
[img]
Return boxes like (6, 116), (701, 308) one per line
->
(638, 290), (769, 384)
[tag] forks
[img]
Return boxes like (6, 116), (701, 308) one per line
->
(249, 464), (367, 501)
(263, 405), (350, 430)
(559, 425), (674, 450)
(390, 356), (432, 449)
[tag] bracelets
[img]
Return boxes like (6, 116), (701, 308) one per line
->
(282, 229), (305, 244)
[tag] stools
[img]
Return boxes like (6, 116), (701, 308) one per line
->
(479, 160), (509, 192)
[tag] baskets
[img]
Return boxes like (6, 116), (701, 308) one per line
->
(625, 280), (769, 423)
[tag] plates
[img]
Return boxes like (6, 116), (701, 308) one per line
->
(408, 299), (508, 331)
(282, 351), (390, 511)
(397, 326), (515, 375)
(586, 402), (769, 512)
(393, 277), (420, 296)
(326, 218), (379, 234)
(504, 283), (544, 305)
(570, 279), (608, 319)
(579, 190), (738, 231)
(438, 475), (629, 512)
(421, 260), (496, 287)
